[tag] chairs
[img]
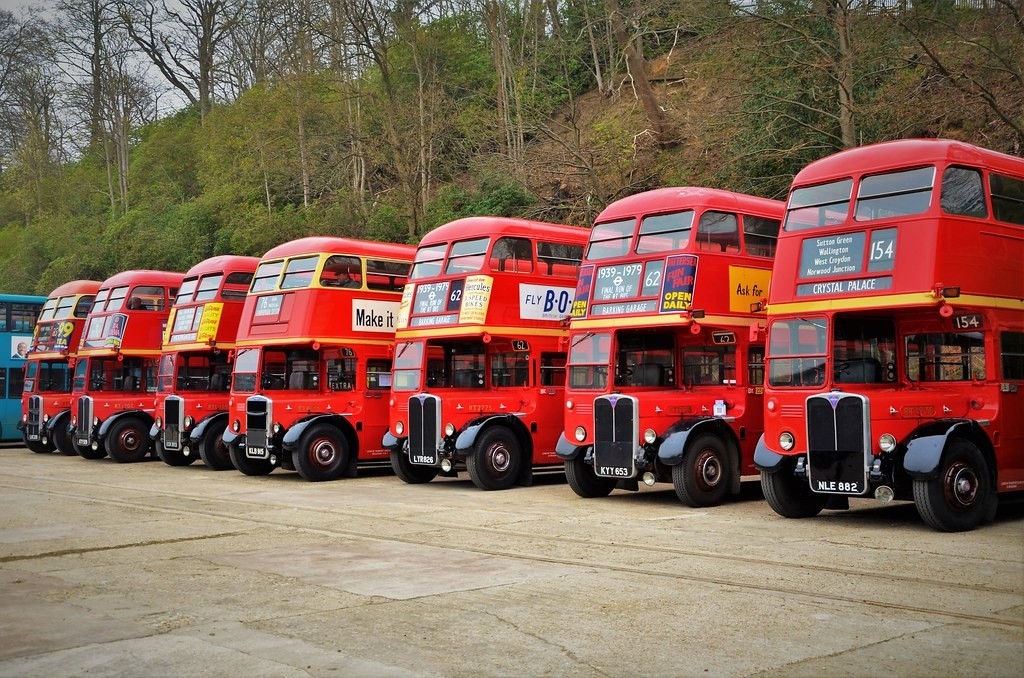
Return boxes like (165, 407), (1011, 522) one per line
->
(631, 363), (664, 387)
(16, 321), (30, 331)
(454, 368), (479, 388)
(123, 376), (137, 391)
(289, 371), (310, 390)
(0, 319), (12, 330)
(841, 358), (883, 383)
(211, 373), (228, 391)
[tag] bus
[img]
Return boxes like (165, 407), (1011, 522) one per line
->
(0, 236), (578, 482)
(381, 216), (744, 491)
(555, 187), (934, 508)
(753, 137), (1024, 533)
